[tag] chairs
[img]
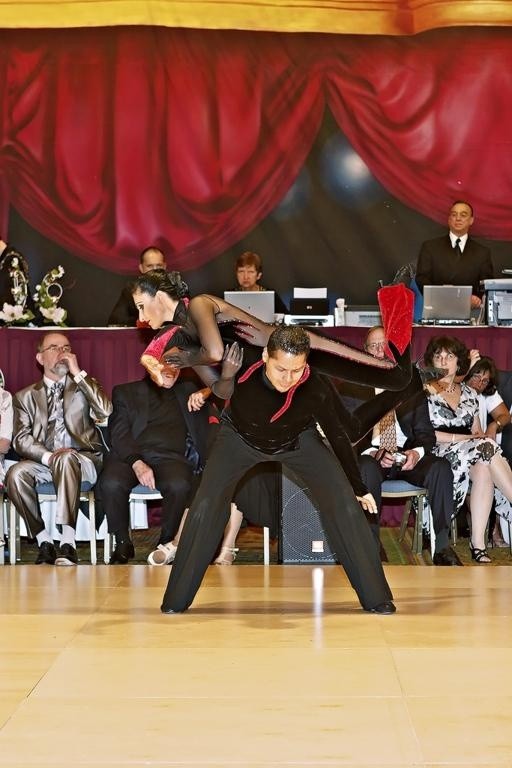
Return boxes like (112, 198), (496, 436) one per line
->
(0, 458), (278, 566)
(375, 479), (430, 557)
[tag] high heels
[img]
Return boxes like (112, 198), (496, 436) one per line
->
(469, 540), (493, 563)
(377, 263), (415, 289)
(414, 354), (448, 384)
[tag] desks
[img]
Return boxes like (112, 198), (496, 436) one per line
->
(0, 315), (511, 414)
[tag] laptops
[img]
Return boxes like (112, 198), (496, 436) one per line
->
(422, 286), (473, 320)
(224, 291), (275, 324)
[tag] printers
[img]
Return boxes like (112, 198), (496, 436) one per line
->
(283, 286), (334, 327)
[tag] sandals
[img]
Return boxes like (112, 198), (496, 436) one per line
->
(214, 547), (239, 565)
(147, 542), (177, 565)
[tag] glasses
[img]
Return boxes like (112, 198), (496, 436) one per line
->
(40, 343), (72, 353)
(366, 342), (384, 350)
(473, 375), (489, 387)
(433, 353), (456, 362)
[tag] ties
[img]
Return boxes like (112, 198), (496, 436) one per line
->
(379, 409), (397, 454)
(455, 238), (461, 256)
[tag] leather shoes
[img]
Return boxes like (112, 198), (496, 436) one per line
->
(55, 544), (78, 565)
(433, 548), (463, 566)
(161, 607), (184, 614)
(372, 601), (396, 613)
(109, 541), (135, 564)
(35, 541), (56, 565)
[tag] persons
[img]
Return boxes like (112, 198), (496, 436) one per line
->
(417, 201), (494, 310)
(108, 246), (167, 326)
(3, 332), (277, 567)
(139, 320), (401, 619)
(130, 263), (415, 400)
(0, 239), (35, 327)
(356, 325), (511, 566)
(228, 251), (289, 313)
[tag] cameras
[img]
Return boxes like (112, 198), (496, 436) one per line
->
(391, 452), (407, 472)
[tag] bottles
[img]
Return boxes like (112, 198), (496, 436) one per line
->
(55, 358), (70, 377)
